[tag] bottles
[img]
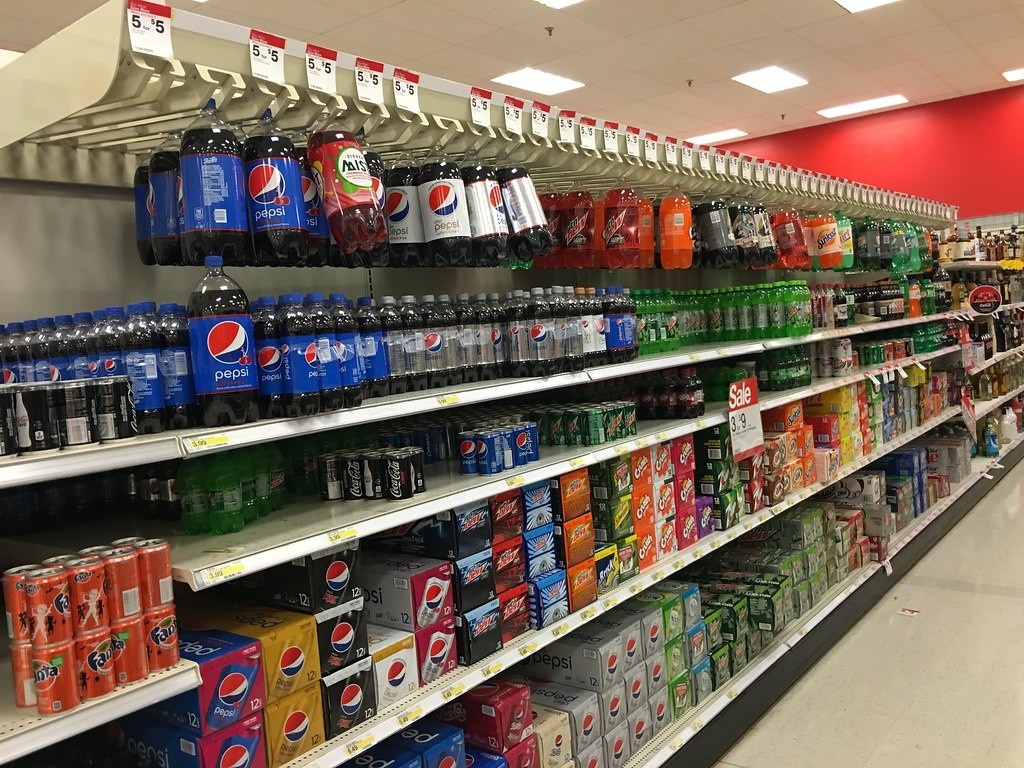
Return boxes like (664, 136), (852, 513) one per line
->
(0, 97), (1024, 534)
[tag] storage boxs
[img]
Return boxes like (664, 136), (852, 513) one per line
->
(414, 613), (458, 687)
(454, 361), (973, 768)
(320, 656), (378, 741)
(99, 710), (267, 768)
(312, 551), (454, 633)
(367, 623), (420, 711)
(385, 717), (466, 768)
(263, 681), (325, 768)
(340, 742), (422, 768)
(126, 629), (266, 738)
(432, 680), (534, 756)
(175, 604), (321, 706)
(261, 597), (370, 677)
(366, 498), (493, 562)
(215, 539), (365, 615)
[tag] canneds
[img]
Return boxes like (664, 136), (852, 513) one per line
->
(316, 400), (637, 502)
(0, 374), (139, 461)
(3, 536), (181, 717)
(805, 336), (914, 378)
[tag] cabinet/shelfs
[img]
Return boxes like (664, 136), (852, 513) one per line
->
(940, 261), (1024, 511)
(0, 0), (969, 768)
